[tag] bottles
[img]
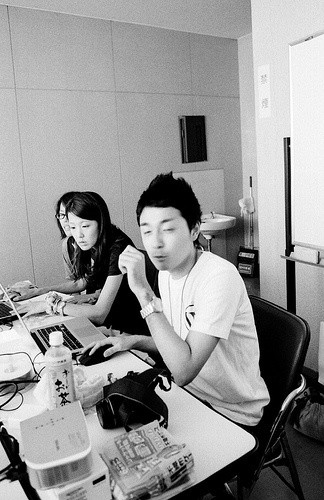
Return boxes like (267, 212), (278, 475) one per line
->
(44, 331), (76, 408)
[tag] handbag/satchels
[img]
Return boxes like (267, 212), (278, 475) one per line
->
(288, 386), (324, 441)
(96, 367), (171, 434)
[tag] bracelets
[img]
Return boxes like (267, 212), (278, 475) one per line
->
(52, 299), (61, 314)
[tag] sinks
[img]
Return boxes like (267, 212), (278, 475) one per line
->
(198, 214), (236, 230)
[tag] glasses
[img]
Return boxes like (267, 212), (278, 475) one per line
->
(55, 213), (67, 219)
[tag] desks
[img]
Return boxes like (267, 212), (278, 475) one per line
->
(0, 291), (261, 500)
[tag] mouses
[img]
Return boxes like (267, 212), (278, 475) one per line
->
(3, 291), (21, 301)
(79, 344), (114, 367)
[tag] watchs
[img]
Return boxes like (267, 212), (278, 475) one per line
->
(140, 297), (163, 319)
(59, 301), (66, 317)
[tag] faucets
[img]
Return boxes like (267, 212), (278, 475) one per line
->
(211, 212), (215, 219)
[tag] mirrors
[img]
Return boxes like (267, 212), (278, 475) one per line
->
(179, 115), (207, 163)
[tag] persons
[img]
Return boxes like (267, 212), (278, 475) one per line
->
(3, 191), (140, 337)
(55, 192), (82, 295)
(78, 172), (270, 500)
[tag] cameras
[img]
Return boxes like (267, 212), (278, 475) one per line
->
(96, 371), (168, 429)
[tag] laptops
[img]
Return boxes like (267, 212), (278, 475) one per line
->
(0, 302), (26, 326)
(0, 284), (107, 355)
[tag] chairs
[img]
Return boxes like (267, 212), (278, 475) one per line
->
(203, 295), (310, 499)
